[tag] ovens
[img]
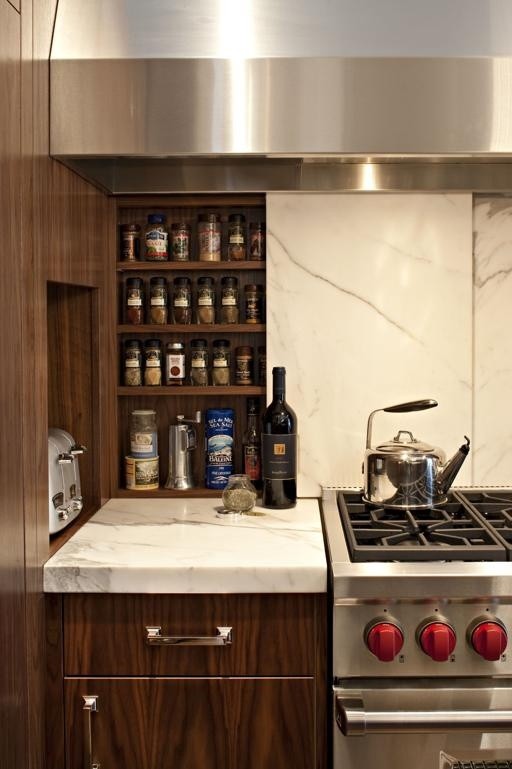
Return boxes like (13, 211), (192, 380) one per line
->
(331, 684), (512, 769)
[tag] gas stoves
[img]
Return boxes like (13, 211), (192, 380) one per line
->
(320, 484), (512, 682)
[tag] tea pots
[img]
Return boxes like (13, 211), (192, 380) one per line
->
(360, 399), (469, 509)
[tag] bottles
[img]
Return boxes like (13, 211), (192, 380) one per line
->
(197, 276), (215, 324)
(205, 408), (233, 489)
(123, 338), (143, 386)
(244, 284), (261, 324)
(235, 346), (253, 386)
(149, 276), (168, 324)
(188, 338), (209, 385)
(122, 223), (141, 261)
(172, 277), (192, 324)
(145, 213), (169, 260)
(257, 345), (265, 386)
(248, 222), (265, 260)
(242, 399), (260, 489)
(165, 342), (186, 386)
(260, 367), (297, 509)
(196, 212), (222, 261)
(144, 339), (162, 386)
(125, 277), (146, 325)
(212, 339), (231, 385)
(163, 411), (202, 492)
(220, 276), (239, 324)
(170, 222), (191, 262)
(227, 213), (246, 260)
(221, 473), (257, 512)
(121, 407), (160, 493)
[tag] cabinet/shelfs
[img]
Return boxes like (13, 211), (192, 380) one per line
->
(47, 593), (327, 768)
(106, 192), (267, 499)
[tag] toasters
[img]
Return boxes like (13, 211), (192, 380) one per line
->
(48, 428), (87, 533)
